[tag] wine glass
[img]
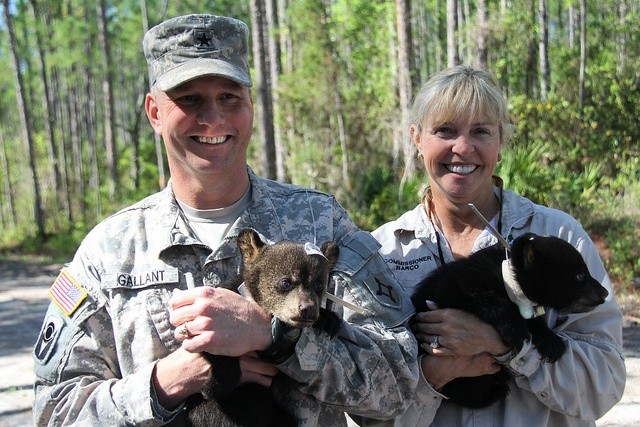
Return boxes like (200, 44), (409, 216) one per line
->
(179, 324), (190, 339)
(428, 337), (441, 349)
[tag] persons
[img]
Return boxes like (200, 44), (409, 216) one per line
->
(364, 66), (623, 426)
(31, 15), (421, 427)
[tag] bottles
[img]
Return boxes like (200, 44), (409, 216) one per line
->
(142, 14), (253, 92)
